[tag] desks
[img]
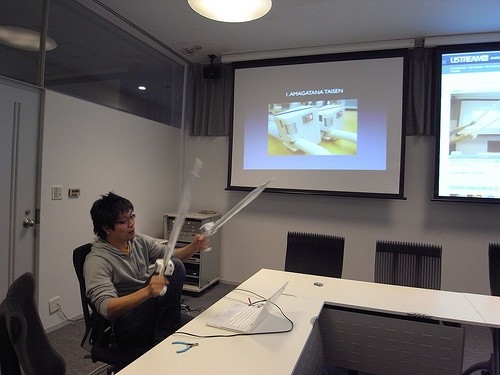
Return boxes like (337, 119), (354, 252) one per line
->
(116, 267), (500, 375)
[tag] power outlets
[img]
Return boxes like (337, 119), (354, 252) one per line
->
(52, 186), (63, 199)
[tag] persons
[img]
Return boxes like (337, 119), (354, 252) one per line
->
(83, 191), (209, 354)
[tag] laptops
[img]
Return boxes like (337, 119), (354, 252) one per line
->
(206, 280), (290, 333)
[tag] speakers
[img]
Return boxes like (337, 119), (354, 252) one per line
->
(203, 65), (222, 79)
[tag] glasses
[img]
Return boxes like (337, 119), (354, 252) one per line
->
(115, 214), (136, 224)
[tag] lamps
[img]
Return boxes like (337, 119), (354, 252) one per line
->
(0, 24), (57, 53)
(187, 0), (272, 26)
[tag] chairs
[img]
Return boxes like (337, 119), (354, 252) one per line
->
(462, 243), (500, 375)
(0, 275), (67, 375)
(375, 239), (442, 289)
(73, 242), (194, 347)
(285, 232), (345, 276)
